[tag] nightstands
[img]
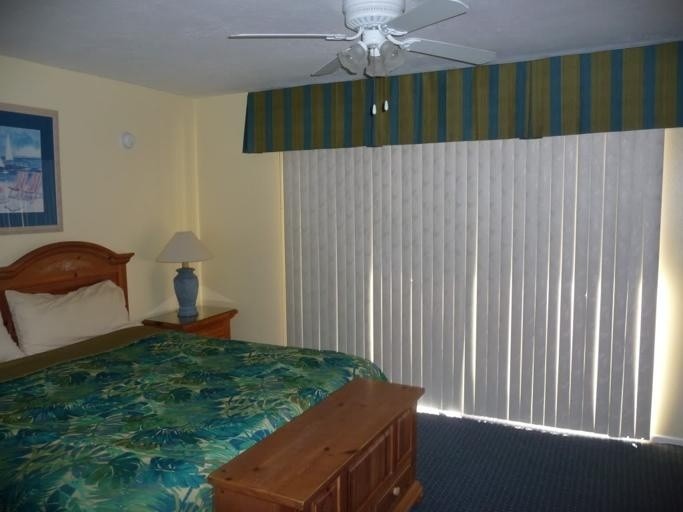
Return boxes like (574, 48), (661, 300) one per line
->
(140, 303), (237, 340)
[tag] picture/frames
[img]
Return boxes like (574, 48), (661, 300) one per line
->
(0, 101), (63, 234)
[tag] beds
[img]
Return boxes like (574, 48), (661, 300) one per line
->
(0, 240), (388, 512)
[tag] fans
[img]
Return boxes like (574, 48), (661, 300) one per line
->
(225, 0), (497, 78)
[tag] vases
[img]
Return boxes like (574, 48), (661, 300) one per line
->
(155, 231), (216, 318)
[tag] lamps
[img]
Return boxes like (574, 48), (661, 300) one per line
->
(336, 29), (405, 78)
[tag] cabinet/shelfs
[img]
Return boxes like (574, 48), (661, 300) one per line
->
(205, 376), (424, 510)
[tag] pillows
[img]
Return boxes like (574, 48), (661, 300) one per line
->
(0, 310), (25, 363)
(5, 280), (129, 357)
